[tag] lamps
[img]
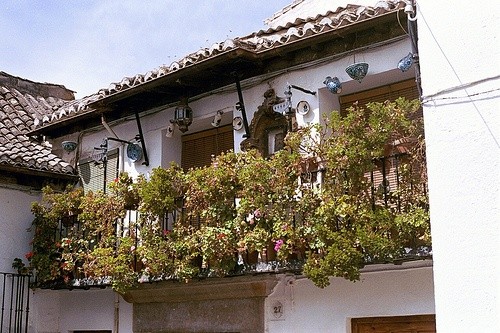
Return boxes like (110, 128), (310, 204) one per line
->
(174, 82), (193, 132)
(397, 52), (415, 72)
(166, 119), (176, 138)
(211, 110), (223, 128)
(323, 76), (342, 94)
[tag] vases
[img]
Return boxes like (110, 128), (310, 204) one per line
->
(61, 215), (76, 227)
(393, 137), (418, 154)
(131, 261), (146, 273)
(241, 250), (258, 263)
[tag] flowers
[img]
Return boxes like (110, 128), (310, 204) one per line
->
(12, 96), (434, 293)
